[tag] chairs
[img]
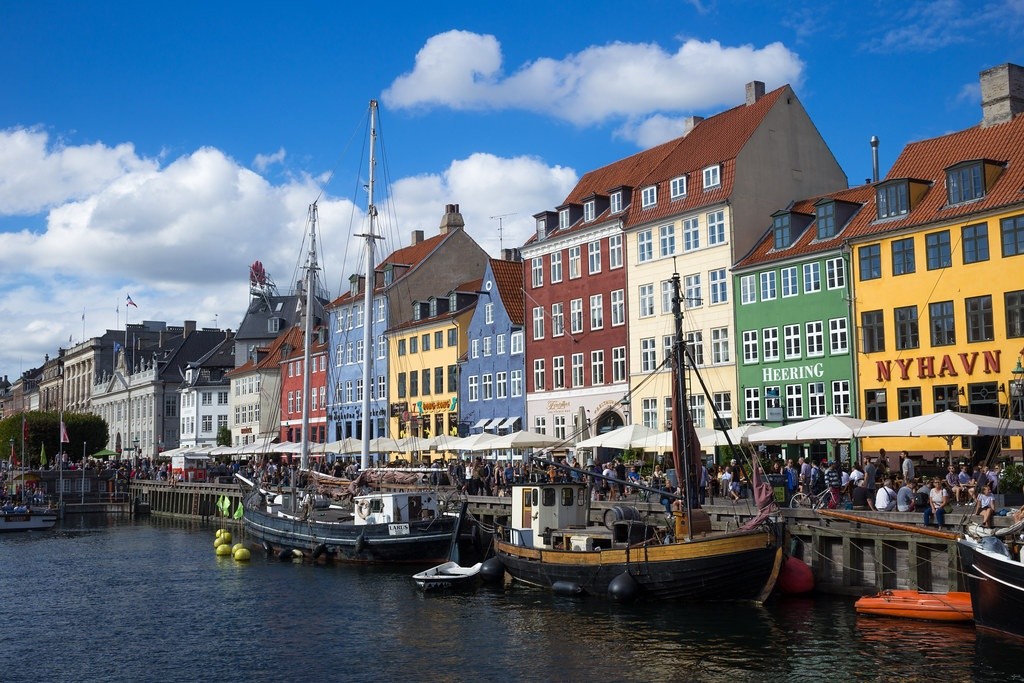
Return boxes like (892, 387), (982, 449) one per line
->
(945, 482), (966, 505)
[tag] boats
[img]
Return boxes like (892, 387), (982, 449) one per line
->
(956, 365), (1024, 638)
(412, 561), (483, 594)
(0, 506), (58, 531)
(855, 587), (972, 621)
(490, 258), (785, 603)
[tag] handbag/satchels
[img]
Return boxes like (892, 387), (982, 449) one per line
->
(943, 504), (953, 514)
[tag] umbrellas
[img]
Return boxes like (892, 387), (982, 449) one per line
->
(577, 423), (774, 462)
(575, 406), (591, 465)
(437, 430), (573, 462)
(747, 411), (883, 462)
(157, 434), (463, 463)
(853, 410), (1024, 473)
(92, 449), (120, 456)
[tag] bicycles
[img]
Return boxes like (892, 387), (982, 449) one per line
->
(790, 480), (845, 509)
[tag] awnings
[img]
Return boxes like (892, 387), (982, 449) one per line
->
(473, 417), (519, 429)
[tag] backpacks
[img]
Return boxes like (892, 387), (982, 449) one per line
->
(912, 493), (928, 512)
(814, 467), (825, 484)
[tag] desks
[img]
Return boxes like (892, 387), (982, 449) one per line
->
(961, 483), (976, 487)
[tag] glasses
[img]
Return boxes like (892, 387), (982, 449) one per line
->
(788, 462), (794, 464)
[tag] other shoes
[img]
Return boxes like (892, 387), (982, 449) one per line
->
(667, 513), (673, 519)
(734, 497), (739, 504)
(971, 501), (977, 507)
(957, 503), (962, 508)
(938, 526), (941, 532)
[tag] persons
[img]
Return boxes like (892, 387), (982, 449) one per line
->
(393, 455), (680, 519)
(308, 459), (361, 481)
(699, 448), (1007, 531)
(206, 458), (301, 486)
(0, 452), (172, 513)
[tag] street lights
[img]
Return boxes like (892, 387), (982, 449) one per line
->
(9, 438), (15, 466)
(132, 437), (140, 478)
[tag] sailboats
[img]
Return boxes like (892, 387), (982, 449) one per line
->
(239, 99), (476, 562)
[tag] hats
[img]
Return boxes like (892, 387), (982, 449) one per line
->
(730, 459), (737, 463)
(827, 460), (836, 466)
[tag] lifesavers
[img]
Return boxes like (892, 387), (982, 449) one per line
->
(357, 500), (371, 520)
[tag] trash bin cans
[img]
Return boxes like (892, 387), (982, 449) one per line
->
(762, 474), (789, 507)
(219, 476), (233, 484)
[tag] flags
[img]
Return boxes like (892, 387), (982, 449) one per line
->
(40, 446), (47, 465)
(60, 422), (69, 442)
(24, 419), (28, 439)
(114, 342), (122, 353)
(126, 296), (137, 307)
(12, 448), (17, 465)
